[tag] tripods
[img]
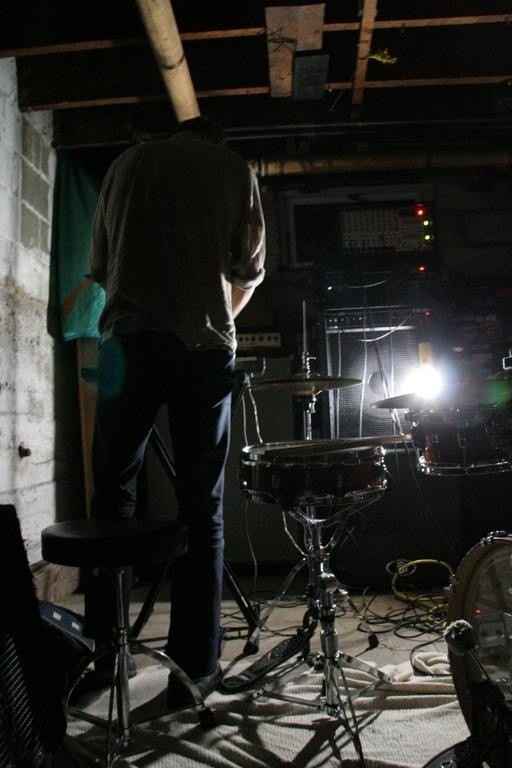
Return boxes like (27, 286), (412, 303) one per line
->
(243, 394), (378, 656)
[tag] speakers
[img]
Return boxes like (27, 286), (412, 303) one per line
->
(324, 326), (424, 457)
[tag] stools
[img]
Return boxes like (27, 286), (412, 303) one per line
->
(43, 521), (210, 768)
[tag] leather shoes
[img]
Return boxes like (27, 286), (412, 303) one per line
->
(167, 659), (224, 709)
(93, 650), (140, 683)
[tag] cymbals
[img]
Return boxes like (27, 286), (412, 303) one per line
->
(266, 428), (409, 455)
(248, 374), (360, 391)
(372, 387), (461, 411)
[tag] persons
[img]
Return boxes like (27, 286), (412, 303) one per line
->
(83, 115), (265, 707)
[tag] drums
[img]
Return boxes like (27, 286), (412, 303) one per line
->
(403, 377), (512, 476)
(240, 440), (390, 509)
(443, 529), (512, 766)
